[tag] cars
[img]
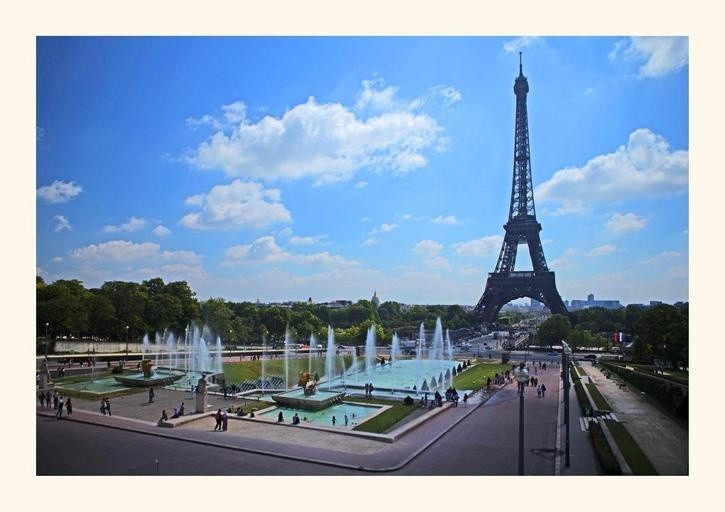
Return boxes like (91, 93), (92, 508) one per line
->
(583, 354), (598, 359)
(285, 341), (348, 349)
(386, 338), (451, 353)
(547, 350), (558, 356)
(458, 328), (527, 350)
(54, 334), (110, 342)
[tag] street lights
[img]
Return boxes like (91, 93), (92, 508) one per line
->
(124, 325), (130, 361)
(316, 332), (321, 358)
(560, 338), (572, 425)
(563, 346), (573, 469)
(513, 359), (531, 475)
(293, 331), (298, 357)
(264, 330), (270, 357)
(524, 340), (529, 362)
(229, 329), (233, 359)
(44, 321), (49, 358)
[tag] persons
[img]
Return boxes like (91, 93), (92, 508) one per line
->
(36, 373), (40, 385)
(527, 377), (546, 398)
(39, 390), (72, 419)
(419, 386), (467, 408)
(149, 388), (154, 403)
(160, 410), (167, 421)
(80, 357), (96, 368)
(103, 397), (111, 416)
(369, 383), (373, 396)
(365, 383), (369, 397)
(293, 413), (300, 424)
(137, 362), (140, 371)
(179, 402), (184, 415)
(56, 366), (64, 378)
(214, 407), (255, 431)
(170, 409), (178, 419)
(278, 412), (284, 422)
(100, 398), (106, 414)
